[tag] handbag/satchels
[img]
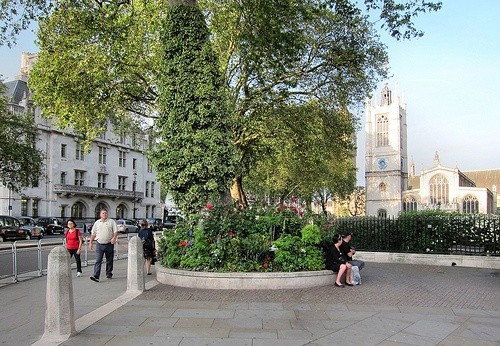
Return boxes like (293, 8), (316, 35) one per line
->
(351, 265), (362, 285)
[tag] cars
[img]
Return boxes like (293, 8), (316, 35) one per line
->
(14, 215), (46, 240)
(0, 214), (32, 244)
(87, 213), (181, 235)
(33, 216), (64, 236)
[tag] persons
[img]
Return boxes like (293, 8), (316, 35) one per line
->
(326, 232), (353, 288)
(65, 219), (82, 277)
(89, 208), (118, 282)
(339, 231), (364, 285)
(138, 220), (154, 275)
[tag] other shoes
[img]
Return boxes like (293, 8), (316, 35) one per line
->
(77, 272), (82, 277)
(147, 273), (152, 275)
(90, 276), (99, 282)
(106, 274), (112, 278)
(345, 281), (353, 287)
(335, 282), (345, 288)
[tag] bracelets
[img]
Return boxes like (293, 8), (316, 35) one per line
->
(79, 248), (81, 250)
